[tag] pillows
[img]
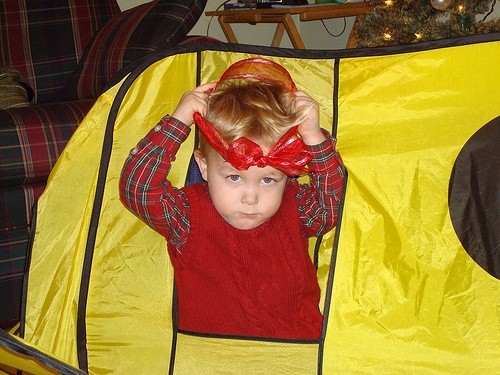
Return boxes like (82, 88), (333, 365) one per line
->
(57, 0), (211, 102)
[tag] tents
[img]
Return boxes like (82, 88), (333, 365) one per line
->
(0, 37), (500, 374)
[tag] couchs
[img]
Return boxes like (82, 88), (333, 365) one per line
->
(1, 1), (224, 326)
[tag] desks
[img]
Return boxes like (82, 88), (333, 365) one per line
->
(203, 4), (390, 51)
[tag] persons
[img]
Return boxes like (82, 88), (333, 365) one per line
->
(116, 81), (348, 346)
